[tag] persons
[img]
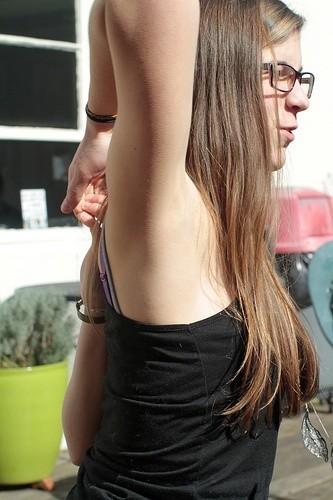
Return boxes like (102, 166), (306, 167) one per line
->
(54, 0), (321, 499)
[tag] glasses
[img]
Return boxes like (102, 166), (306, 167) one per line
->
(257, 60), (316, 99)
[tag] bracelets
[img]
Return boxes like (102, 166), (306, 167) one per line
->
(86, 103), (118, 123)
(76, 300), (107, 325)
